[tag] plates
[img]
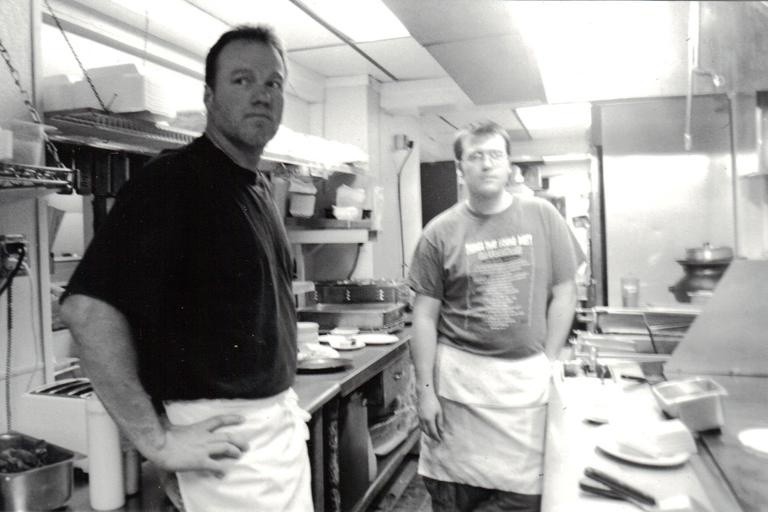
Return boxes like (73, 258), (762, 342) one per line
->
(595, 439), (691, 466)
(298, 333), (400, 370)
(73, 64), (177, 122)
(737, 428), (767, 454)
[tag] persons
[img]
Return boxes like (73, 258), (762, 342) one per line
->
(58, 26), (316, 512)
(403, 123), (588, 512)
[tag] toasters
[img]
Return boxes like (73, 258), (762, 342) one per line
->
(11, 377), (94, 455)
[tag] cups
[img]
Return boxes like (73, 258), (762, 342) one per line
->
(621, 274), (638, 308)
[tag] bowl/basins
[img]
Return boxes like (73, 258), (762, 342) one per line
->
(675, 260), (730, 276)
